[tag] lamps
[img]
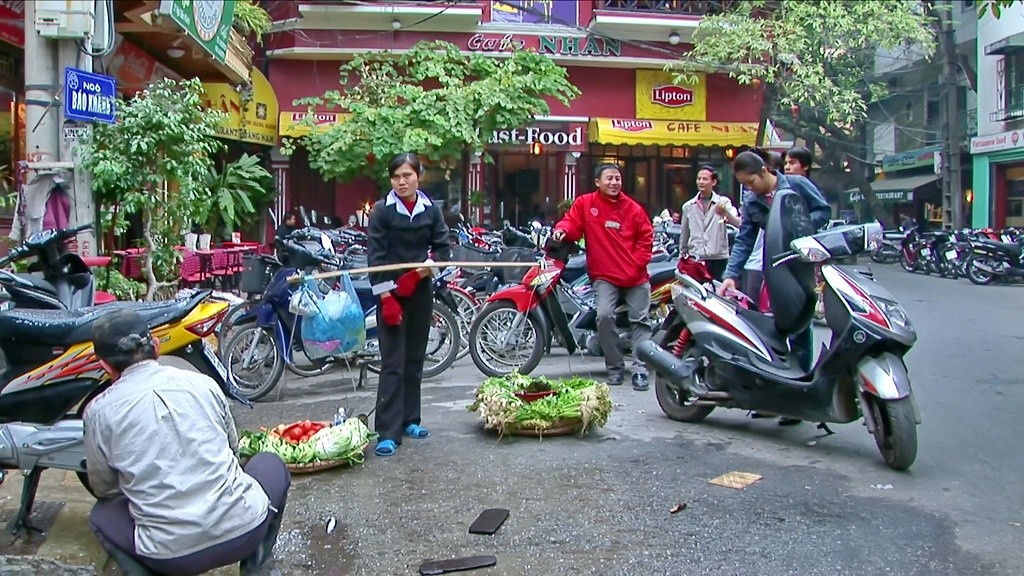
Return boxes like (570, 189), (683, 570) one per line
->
(391, 17), (401, 30)
(166, 47), (186, 58)
(668, 29), (680, 45)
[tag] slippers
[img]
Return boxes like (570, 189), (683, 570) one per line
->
(405, 424), (430, 439)
(376, 439), (397, 456)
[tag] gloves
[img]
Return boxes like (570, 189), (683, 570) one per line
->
(395, 270), (421, 296)
(382, 294), (403, 326)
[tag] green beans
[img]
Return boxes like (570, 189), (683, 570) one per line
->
(263, 425), (354, 466)
(340, 418), (370, 465)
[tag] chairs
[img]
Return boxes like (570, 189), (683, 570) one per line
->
(109, 244), (273, 292)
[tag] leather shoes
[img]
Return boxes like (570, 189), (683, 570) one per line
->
(631, 372), (648, 391)
(605, 373), (623, 385)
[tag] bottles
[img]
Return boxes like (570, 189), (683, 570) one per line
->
(332, 407), (348, 427)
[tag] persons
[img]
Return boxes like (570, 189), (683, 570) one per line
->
(679, 165), (741, 283)
(719, 146), (832, 426)
(781, 146), (827, 203)
(274, 212), (296, 237)
(737, 147), (772, 312)
(899, 213), (911, 231)
(659, 208), (680, 225)
(552, 163), (654, 391)
(83, 309), (291, 576)
(368, 152), (449, 456)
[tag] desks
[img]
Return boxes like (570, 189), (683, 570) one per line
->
(222, 240), (258, 258)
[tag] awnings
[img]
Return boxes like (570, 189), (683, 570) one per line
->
(278, 109), (371, 138)
(588, 116), (771, 150)
(843, 175), (943, 204)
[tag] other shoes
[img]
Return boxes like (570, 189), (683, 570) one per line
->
(778, 416), (801, 426)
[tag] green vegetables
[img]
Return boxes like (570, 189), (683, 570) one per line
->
(240, 428), (265, 459)
(466, 370), (612, 436)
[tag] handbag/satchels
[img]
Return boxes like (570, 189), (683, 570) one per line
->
(299, 269), (368, 360)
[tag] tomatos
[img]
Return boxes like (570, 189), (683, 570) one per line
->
(283, 419), (324, 445)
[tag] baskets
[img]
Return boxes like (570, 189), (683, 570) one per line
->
(504, 415), (580, 434)
(267, 418), (366, 473)
(502, 247), (537, 283)
(239, 255), (277, 295)
(461, 244), (497, 273)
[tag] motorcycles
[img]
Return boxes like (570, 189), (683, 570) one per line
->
(869, 225), (1023, 283)
(631, 219), (920, 471)
(3, 221), (243, 459)
(74, 206), (684, 380)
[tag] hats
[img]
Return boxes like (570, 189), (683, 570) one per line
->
(93, 308), (151, 355)
